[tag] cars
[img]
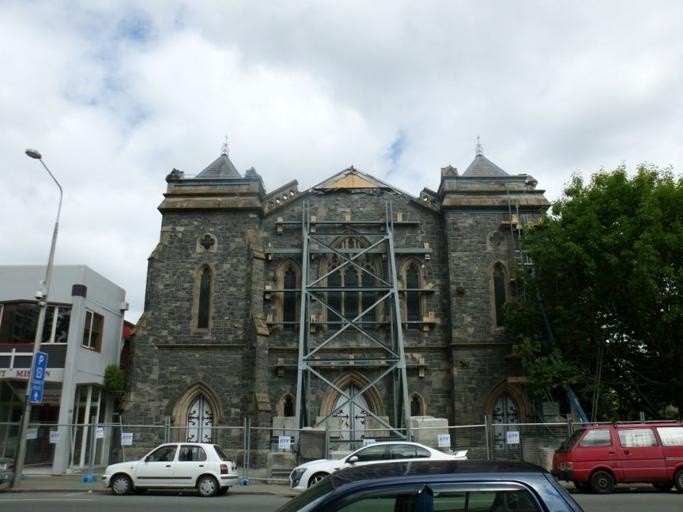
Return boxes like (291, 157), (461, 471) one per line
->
(287, 441), (469, 497)
(271, 460), (585, 512)
(98, 442), (240, 497)
(0, 455), (14, 488)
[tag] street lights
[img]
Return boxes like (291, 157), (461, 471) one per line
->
(11, 148), (65, 493)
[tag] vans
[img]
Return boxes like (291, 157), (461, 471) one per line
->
(548, 420), (683, 492)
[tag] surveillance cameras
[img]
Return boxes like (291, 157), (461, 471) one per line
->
(34, 291), (43, 300)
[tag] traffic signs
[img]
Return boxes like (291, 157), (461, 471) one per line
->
(29, 380), (44, 403)
